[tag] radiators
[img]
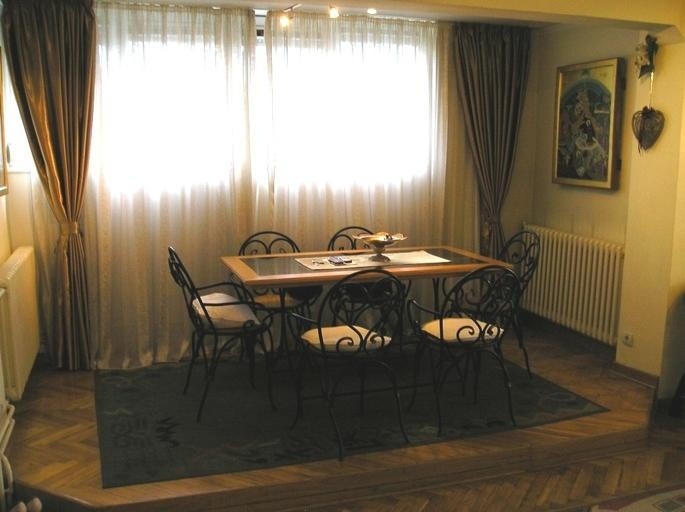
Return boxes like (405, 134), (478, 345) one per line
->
(518, 220), (624, 345)
(0, 245), (40, 402)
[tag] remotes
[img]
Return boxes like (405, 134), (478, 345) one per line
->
(328, 256), (343, 265)
(342, 255), (353, 263)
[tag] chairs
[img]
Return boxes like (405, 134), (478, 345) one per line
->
(446, 229), (540, 380)
(169, 245), (278, 424)
(327, 226), (413, 360)
(238, 231), (322, 368)
(283, 269), (412, 462)
(408, 263), (523, 440)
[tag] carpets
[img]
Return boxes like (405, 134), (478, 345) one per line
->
(93, 330), (612, 489)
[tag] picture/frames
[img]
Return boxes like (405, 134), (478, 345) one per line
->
(0, 42), (9, 196)
(550, 57), (625, 189)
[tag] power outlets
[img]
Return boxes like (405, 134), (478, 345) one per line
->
(623, 334), (634, 347)
